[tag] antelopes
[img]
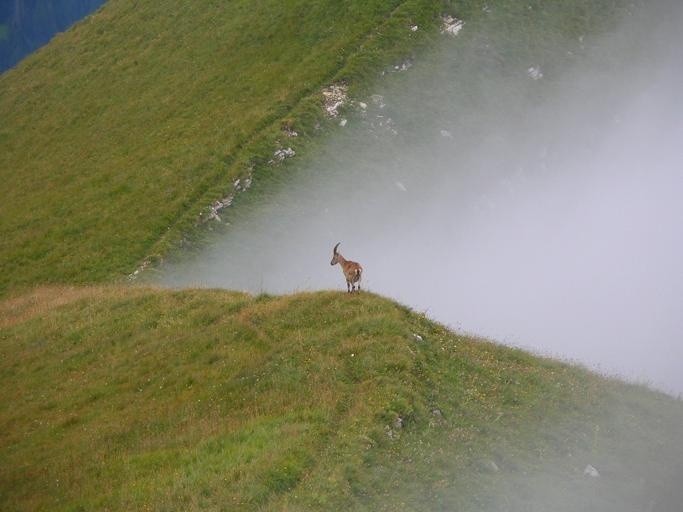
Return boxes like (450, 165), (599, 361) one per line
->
(331, 241), (363, 296)
